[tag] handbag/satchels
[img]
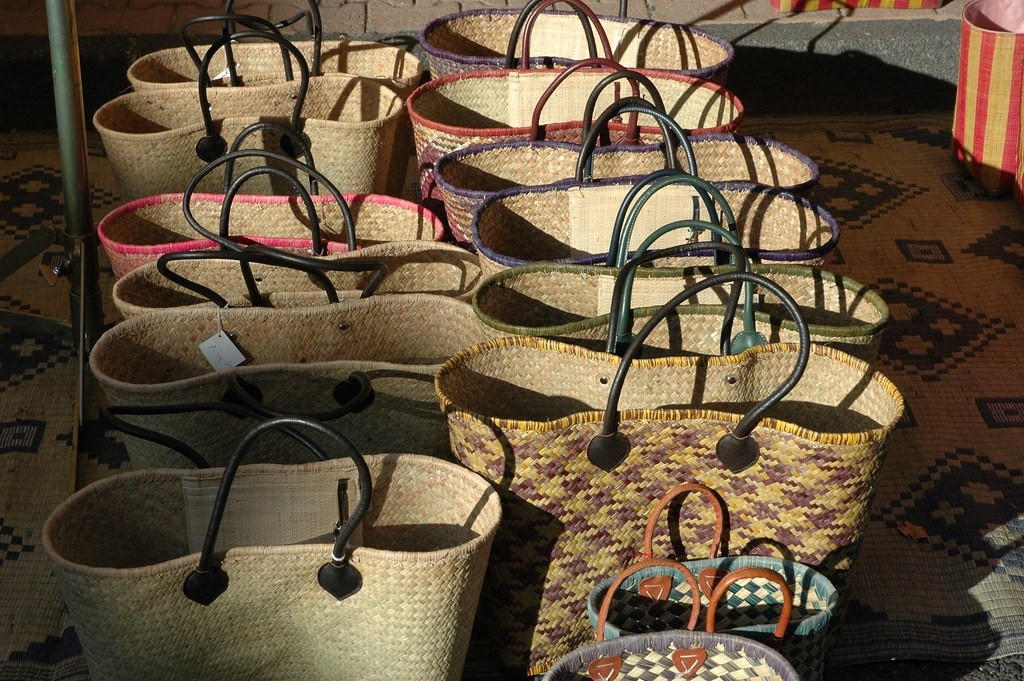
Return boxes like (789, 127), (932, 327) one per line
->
(42, 0), (906, 681)
